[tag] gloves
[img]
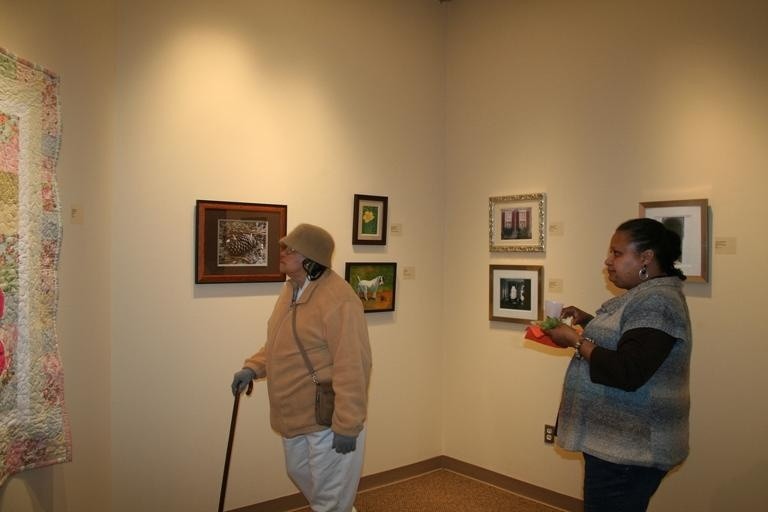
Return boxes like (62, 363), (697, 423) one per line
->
(332, 433), (355, 454)
(231, 368), (255, 395)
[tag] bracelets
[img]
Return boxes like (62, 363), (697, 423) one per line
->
(574, 337), (597, 361)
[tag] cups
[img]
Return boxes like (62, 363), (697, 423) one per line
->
(543, 300), (563, 322)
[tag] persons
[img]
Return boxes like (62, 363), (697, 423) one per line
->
(229, 223), (374, 511)
(543, 217), (692, 512)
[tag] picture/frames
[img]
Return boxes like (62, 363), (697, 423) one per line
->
(196, 200), (287, 284)
(346, 262), (397, 312)
(489, 193), (544, 252)
(489, 265), (543, 324)
(353, 194), (388, 245)
(638, 199), (709, 284)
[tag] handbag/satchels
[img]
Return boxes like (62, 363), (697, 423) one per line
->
(315, 382), (335, 426)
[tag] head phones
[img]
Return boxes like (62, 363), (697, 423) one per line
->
(303, 259), (326, 281)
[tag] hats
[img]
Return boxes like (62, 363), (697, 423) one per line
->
(279, 224), (334, 268)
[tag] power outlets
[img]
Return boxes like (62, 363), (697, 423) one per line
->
(545, 424), (554, 444)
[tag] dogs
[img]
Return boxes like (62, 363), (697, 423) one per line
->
(357, 275), (385, 301)
